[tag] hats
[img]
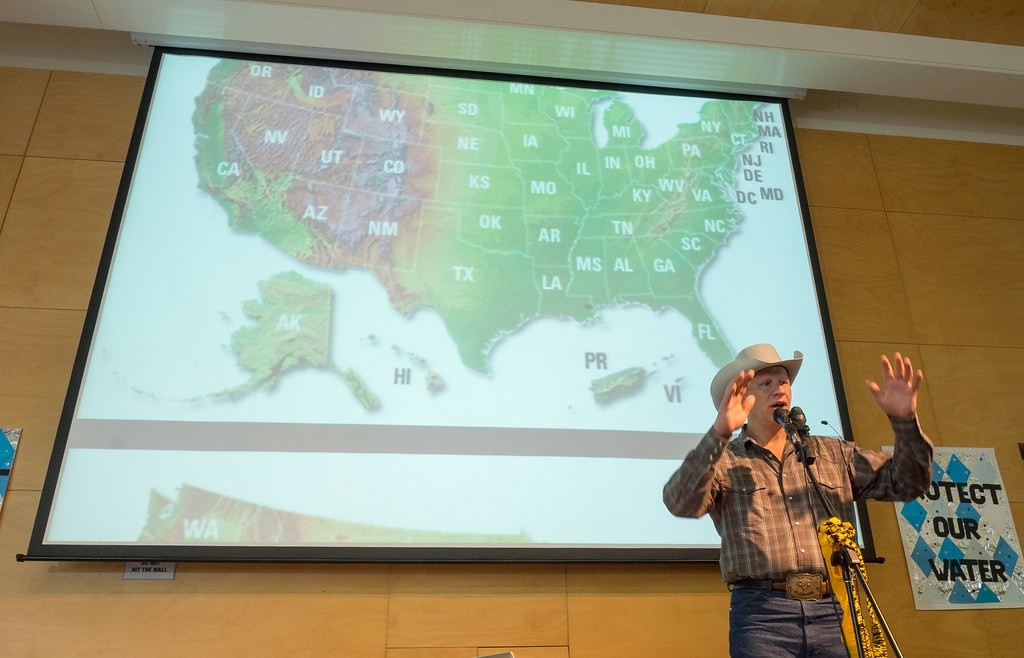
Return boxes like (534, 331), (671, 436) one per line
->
(710, 343), (804, 422)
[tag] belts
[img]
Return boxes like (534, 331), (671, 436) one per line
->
(733, 572), (831, 601)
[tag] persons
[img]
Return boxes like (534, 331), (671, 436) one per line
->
(663, 342), (938, 658)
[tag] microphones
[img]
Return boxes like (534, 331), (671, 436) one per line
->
(821, 420), (828, 426)
(772, 406), (816, 466)
